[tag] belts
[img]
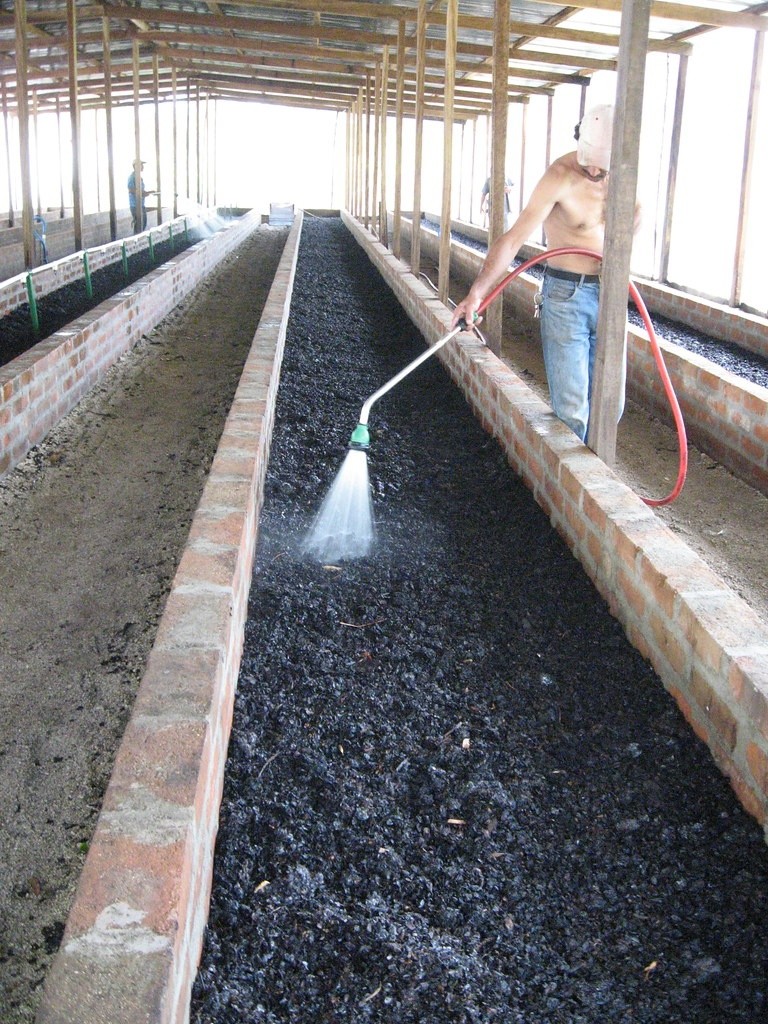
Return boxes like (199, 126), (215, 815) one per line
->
(546, 266), (600, 283)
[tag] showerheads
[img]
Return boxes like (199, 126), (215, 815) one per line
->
(173, 193), (179, 198)
(348, 422), (372, 452)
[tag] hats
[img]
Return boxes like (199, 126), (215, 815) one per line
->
(577, 105), (616, 172)
(132, 159), (146, 167)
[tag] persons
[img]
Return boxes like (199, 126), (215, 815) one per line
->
(479, 170), (514, 232)
(127, 160), (155, 236)
(447, 103), (643, 443)
